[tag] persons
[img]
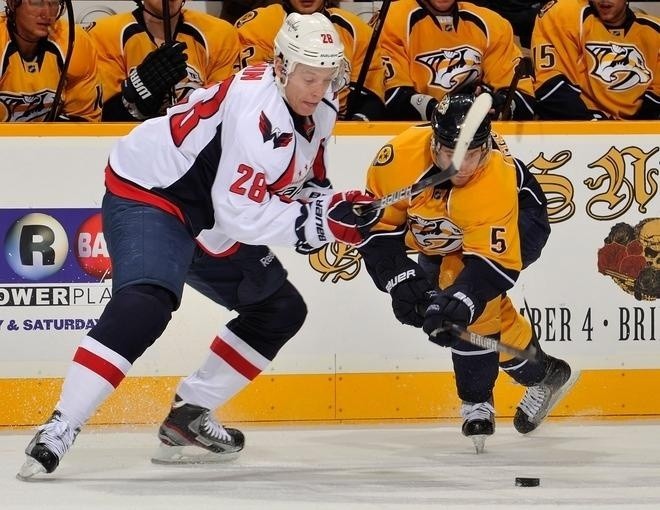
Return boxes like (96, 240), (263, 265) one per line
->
(21, 11), (389, 476)
(360, 91), (570, 437)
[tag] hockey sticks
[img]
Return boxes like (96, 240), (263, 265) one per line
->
(46, 0), (75, 121)
(444, 296), (544, 365)
(351, 91), (492, 215)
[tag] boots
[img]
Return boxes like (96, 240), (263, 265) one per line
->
(157, 391), (245, 454)
(513, 354), (571, 434)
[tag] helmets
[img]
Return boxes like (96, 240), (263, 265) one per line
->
(430, 91), (492, 171)
(270, 10), (345, 89)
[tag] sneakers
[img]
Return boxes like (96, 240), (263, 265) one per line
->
(23, 409), (84, 474)
(460, 391), (496, 438)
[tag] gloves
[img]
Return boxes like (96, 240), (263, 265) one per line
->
(378, 260), (440, 328)
(120, 39), (189, 116)
(304, 188), (386, 249)
(422, 283), (487, 348)
